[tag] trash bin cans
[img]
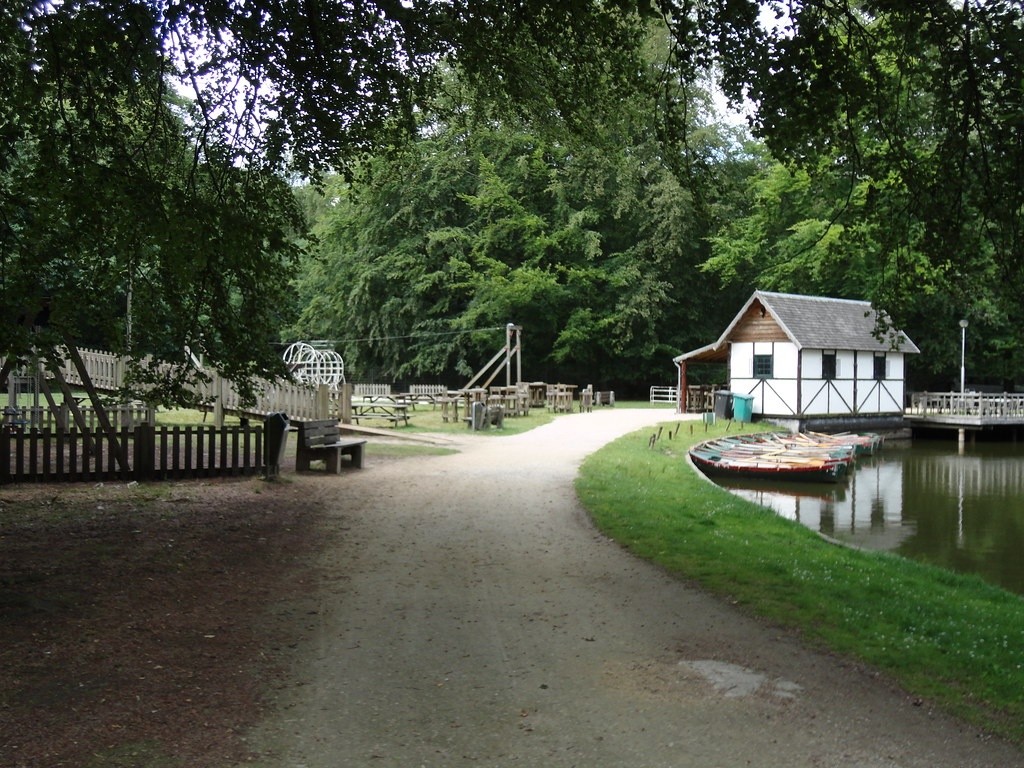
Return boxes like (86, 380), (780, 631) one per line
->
(264, 412), (290, 483)
(470, 402), (487, 430)
(732, 393), (755, 423)
(713, 390), (734, 420)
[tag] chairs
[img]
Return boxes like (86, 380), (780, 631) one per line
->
(441, 382), (593, 421)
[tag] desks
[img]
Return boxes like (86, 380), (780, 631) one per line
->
(400, 392), (441, 410)
(351, 402), (408, 427)
(361, 393), (406, 414)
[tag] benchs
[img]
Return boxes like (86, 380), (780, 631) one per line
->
(291, 419), (367, 474)
(350, 414), (411, 429)
(350, 398), (439, 415)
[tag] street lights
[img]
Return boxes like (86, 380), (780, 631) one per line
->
(959, 319), (968, 396)
